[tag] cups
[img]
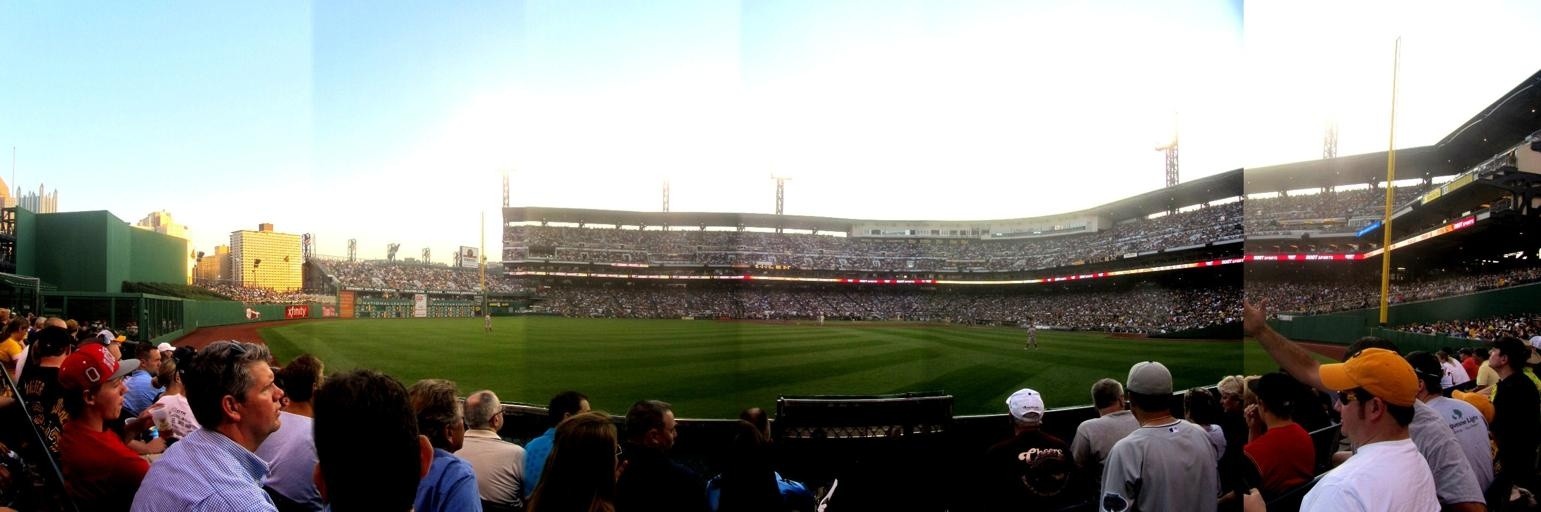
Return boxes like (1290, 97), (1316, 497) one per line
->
(124, 417), (137, 425)
(148, 404), (174, 440)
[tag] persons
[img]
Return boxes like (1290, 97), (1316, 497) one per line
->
(1, 180), (1539, 511)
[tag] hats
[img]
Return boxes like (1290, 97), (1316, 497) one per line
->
(1319, 347), (1420, 406)
(1006, 387), (1045, 424)
(1127, 360), (1174, 395)
(157, 341), (177, 353)
(57, 342), (141, 391)
(1490, 335), (1531, 361)
(1249, 371), (1298, 406)
(1529, 335), (1541, 349)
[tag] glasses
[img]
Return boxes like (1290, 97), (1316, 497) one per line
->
(489, 405), (506, 423)
(1337, 391), (1359, 405)
(615, 444), (623, 457)
